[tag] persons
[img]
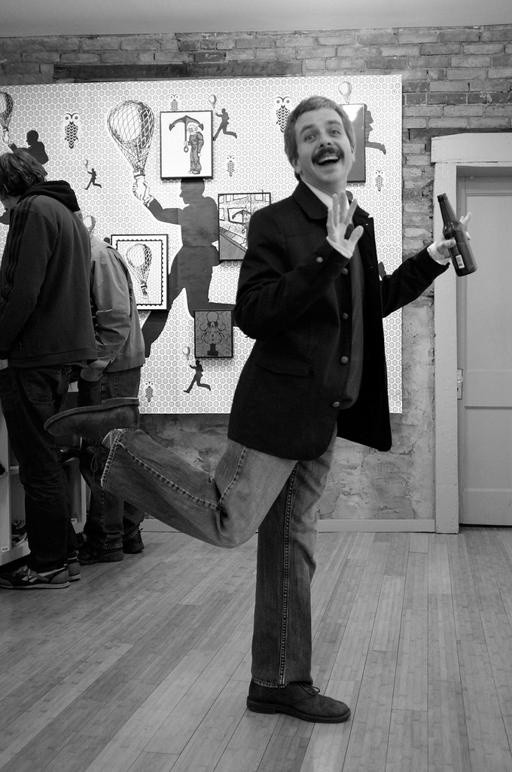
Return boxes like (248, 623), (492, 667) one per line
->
(212, 108), (237, 142)
(0, 130), (50, 226)
(38, 175), (145, 568)
(182, 123), (205, 175)
(183, 360), (211, 397)
(1, 148), (101, 594)
(44, 96), (474, 726)
(127, 180), (239, 360)
(362, 104), (386, 154)
(83, 168), (102, 191)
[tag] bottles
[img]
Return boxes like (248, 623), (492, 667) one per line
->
(437, 193), (478, 277)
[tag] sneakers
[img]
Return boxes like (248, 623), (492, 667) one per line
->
(2, 531), (144, 592)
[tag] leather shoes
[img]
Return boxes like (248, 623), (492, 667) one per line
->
(47, 398), (143, 442)
(246, 679), (351, 722)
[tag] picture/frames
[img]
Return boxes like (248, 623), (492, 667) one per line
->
(340, 103), (367, 182)
(194, 309), (232, 358)
(218, 192), (271, 261)
(160, 112), (213, 180)
(111, 234), (168, 312)
